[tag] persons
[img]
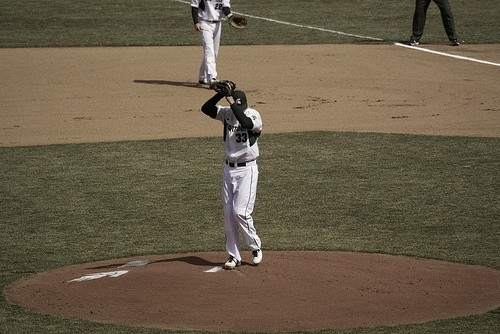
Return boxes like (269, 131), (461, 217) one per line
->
(407, 0), (460, 46)
(189, 0), (248, 88)
(201, 80), (262, 268)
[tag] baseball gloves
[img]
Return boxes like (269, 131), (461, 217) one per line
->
(228, 16), (248, 29)
(209, 78), (237, 99)
